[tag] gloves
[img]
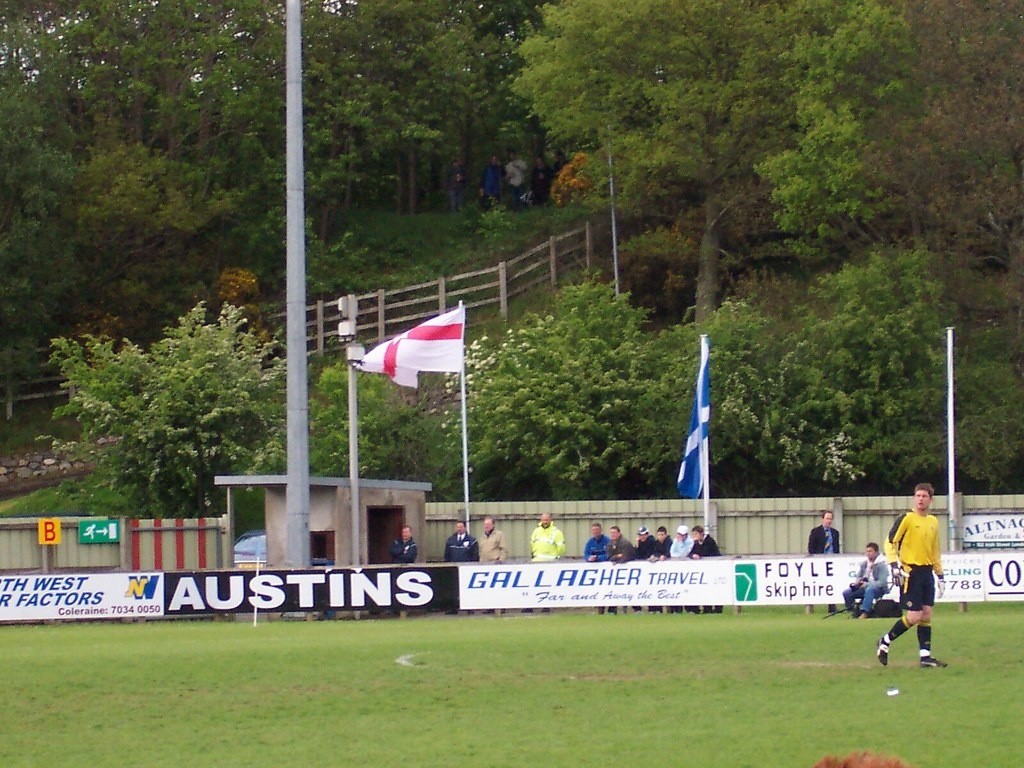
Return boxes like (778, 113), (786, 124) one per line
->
(937, 575), (945, 598)
(890, 561), (910, 586)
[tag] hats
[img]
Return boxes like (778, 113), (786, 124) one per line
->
(637, 527), (648, 535)
(677, 526), (688, 535)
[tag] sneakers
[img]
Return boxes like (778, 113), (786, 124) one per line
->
(921, 657), (948, 667)
(877, 637), (889, 665)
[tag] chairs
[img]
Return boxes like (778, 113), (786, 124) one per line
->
(854, 581), (895, 619)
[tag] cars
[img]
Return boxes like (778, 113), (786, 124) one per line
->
(233, 528), (266, 569)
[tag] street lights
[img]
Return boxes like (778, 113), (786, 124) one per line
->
(336, 294), (366, 564)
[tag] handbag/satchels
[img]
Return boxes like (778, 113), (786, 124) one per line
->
(874, 600), (901, 616)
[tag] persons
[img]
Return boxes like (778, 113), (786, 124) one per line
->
(503, 149), (527, 211)
(632, 526), (723, 614)
(520, 511), (565, 612)
(877, 482), (946, 667)
(442, 521), (478, 614)
(606, 525), (636, 614)
(389, 525), (418, 616)
(478, 518), (507, 615)
(555, 152), (569, 171)
(532, 157), (553, 195)
(808, 510), (840, 614)
(843, 542), (890, 618)
(446, 159), (465, 213)
(480, 156), (505, 210)
(584, 522), (613, 616)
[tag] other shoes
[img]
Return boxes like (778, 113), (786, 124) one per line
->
(859, 612), (871, 618)
(447, 605), (724, 614)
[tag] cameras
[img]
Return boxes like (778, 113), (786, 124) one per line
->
(850, 577), (870, 592)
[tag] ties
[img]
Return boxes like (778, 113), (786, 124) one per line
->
(825, 529), (829, 553)
(459, 536), (462, 542)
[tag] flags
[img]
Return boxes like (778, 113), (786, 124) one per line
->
(675, 335), (711, 499)
(355, 307), (464, 389)
